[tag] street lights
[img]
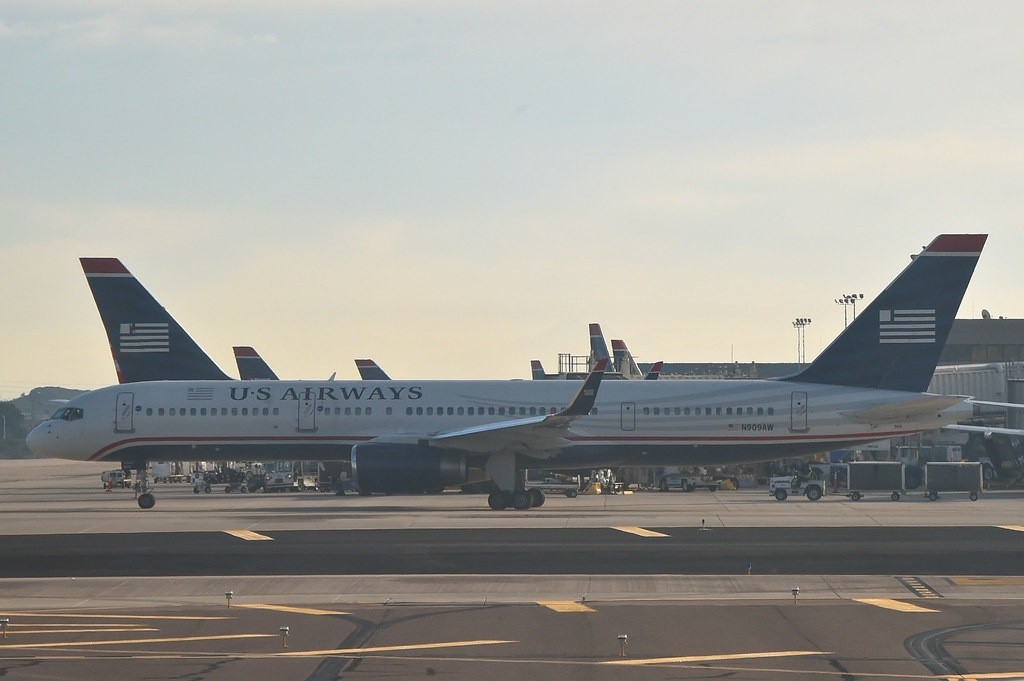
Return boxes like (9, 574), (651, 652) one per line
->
(842, 293), (865, 321)
(792, 321), (806, 372)
(795, 318), (812, 364)
(834, 298), (854, 329)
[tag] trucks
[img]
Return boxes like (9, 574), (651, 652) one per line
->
(656, 465), (722, 492)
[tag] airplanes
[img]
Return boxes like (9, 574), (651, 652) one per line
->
(25, 231), (1023, 512)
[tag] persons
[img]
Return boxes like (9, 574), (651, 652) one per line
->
(793, 461), (813, 488)
